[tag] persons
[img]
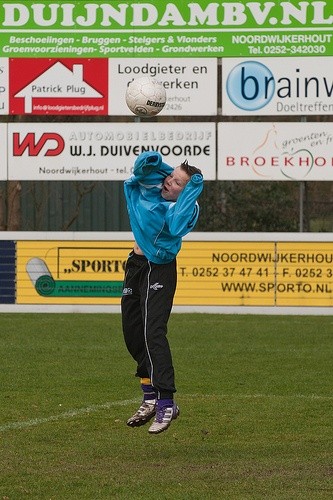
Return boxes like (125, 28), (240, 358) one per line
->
(121, 151), (203, 434)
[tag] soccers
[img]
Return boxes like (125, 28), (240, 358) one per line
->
(125, 75), (168, 118)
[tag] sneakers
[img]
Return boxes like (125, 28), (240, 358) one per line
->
(126, 396), (158, 428)
(147, 403), (181, 435)
